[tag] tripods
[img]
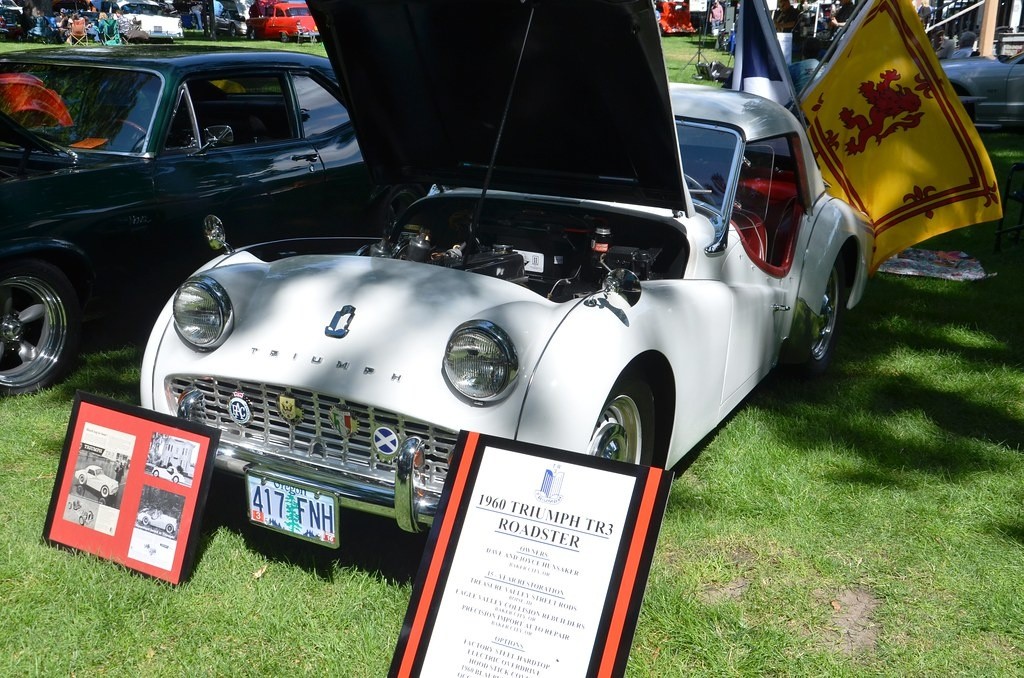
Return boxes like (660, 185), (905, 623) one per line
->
(683, 12), (710, 70)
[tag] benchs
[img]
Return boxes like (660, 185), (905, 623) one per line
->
(678, 145), (797, 262)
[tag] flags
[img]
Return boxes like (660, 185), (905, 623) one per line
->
(799, 0), (1004, 280)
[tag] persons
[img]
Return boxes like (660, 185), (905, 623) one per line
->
(115, 462), (124, 496)
(654, 4), (661, 21)
(788, 37), (826, 97)
(709, 0), (723, 36)
(947, 31), (977, 59)
(917, 1), (931, 21)
(27, 0), (132, 45)
(774, 0), (856, 39)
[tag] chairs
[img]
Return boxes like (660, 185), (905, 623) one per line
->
(17, 16), (141, 46)
(296, 22), (317, 44)
(220, 110), (266, 144)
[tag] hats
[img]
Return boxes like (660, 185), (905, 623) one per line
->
(931, 26), (944, 38)
(961, 31), (977, 41)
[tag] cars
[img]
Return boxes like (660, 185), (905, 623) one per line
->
(0, 40), (404, 402)
(215, 6), (249, 38)
(74, 465), (119, 499)
(244, 2), (322, 44)
(136, 506), (179, 535)
(134, 0), (876, 547)
(0, 0), (184, 43)
(934, 47), (1024, 126)
(151, 465), (185, 485)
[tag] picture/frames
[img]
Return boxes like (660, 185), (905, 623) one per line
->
(40, 389), (221, 588)
